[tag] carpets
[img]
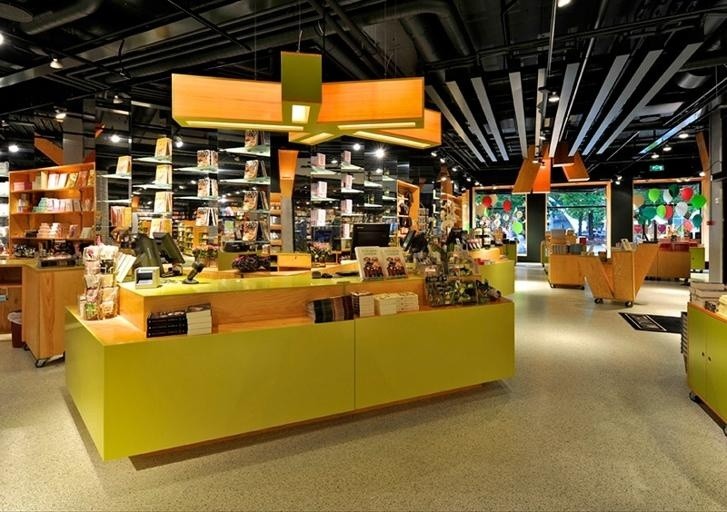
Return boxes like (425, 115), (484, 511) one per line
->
(619, 311), (683, 333)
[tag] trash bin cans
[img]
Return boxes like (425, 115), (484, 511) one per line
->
(7, 310), (25, 348)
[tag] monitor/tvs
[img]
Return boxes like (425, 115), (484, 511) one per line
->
(153, 230), (186, 266)
(402, 230), (417, 249)
(444, 227), (462, 241)
(352, 224), (391, 256)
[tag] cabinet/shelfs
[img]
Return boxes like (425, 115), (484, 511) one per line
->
(540, 240), (585, 290)
(589, 242), (705, 307)
(178, 220), (204, 257)
(397, 180), (421, 236)
(9, 163), (97, 260)
(441, 192), (463, 232)
(686, 301), (727, 432)
(20, 262), (85, 367)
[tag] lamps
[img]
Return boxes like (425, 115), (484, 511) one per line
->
(171, 50), (442, 149)
(431, 149), (480, 187)
(651, 127), (688, 160)
(49, 59), (63, 69)
(549, 91), (559, 102)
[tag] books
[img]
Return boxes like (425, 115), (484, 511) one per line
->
(147, 303), (212, 339)
(109, 155), (133, 232)
(355, 245), (387, 282)
(307, 291), (420, 324)
(688, 281), (727, 306)
(240, 128), (271, 242)
(195, 150), (219, 227)
(381, 247), (410, 280)
(13, 170), (95, 240)
(150, 138), (173, 240)
(341, 150), (353, 238)
(310, 153), (327, 227)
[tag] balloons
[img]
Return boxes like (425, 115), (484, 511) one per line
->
(633, 184), (707, 234)
(476, 194), (524, 245)
